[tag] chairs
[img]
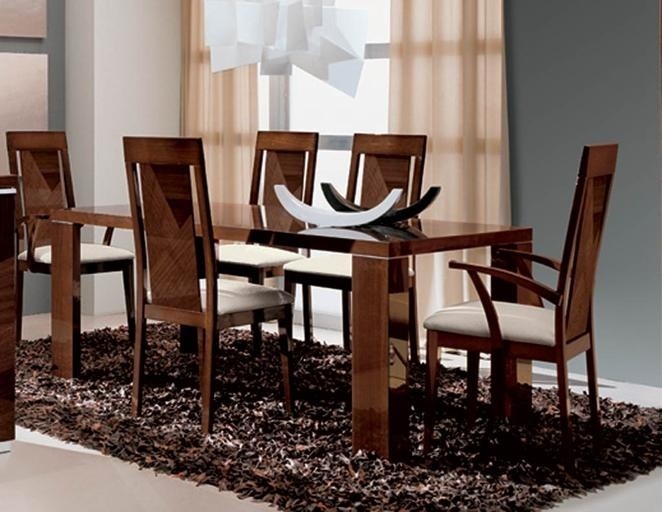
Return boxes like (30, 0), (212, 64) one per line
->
(214, 131), (319, 365)
(282, 133), (427, 365)
(422, 142), (619, 473)
(122, 137), (295, 437)
(6, 131), (136, 380)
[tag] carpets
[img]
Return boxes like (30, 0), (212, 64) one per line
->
(16, 321), (662, 512)
